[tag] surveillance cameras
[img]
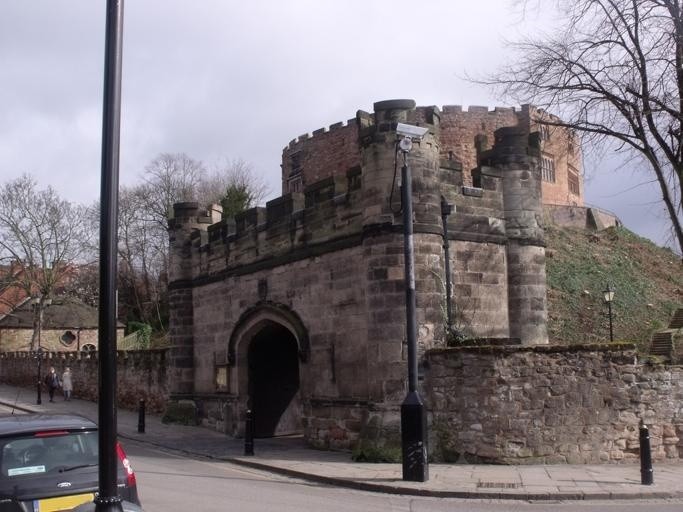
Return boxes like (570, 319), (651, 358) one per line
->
(396, 122), (429, 140)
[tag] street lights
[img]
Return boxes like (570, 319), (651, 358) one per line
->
(601, 283), (615, 342)
(30, 290), (52, 405)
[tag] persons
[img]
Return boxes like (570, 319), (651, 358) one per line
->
(59, 366), (72, 401)
(44, 366), (57, 402)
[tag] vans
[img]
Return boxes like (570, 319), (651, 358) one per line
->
(0, 410), (141, 511)
